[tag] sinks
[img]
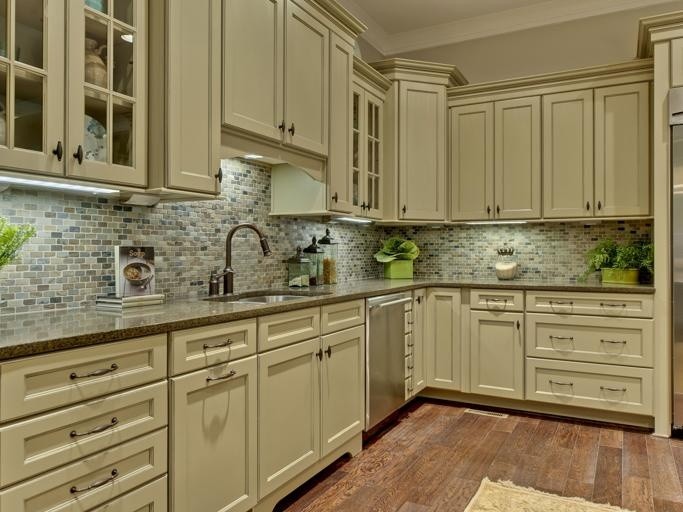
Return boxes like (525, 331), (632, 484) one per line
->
(198, 289), (333, 305)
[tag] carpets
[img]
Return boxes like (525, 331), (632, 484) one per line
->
(458, 472), (637, 512)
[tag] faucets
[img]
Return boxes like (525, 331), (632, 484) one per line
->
(223, 223), (272, 294)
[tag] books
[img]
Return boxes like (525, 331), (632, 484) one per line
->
(94, 293), (164, 309)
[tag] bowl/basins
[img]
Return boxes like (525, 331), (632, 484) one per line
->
(123, 262), (151, 285)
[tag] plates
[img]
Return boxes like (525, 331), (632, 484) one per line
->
(83, 112), (106, 163)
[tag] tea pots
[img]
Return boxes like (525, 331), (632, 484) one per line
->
(83, 37), (109, 87)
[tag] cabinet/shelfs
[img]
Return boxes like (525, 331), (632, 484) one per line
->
(155, 306), (258, 512)
(467, 285), (525, 410)
(519, 281), (652, 433)
(217, 0), (331, 217)
(0, 325), (171, 512)
(1, 0), (150, 193)
(328, 0), (367, 220)
(145, 0), (223, 203)
(404, 277), (469, 404)
(252, 292), (367, 511)
(354, 56), (656, 228)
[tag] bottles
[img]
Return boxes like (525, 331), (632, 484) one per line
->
(302, 237), (324, 287)
(286, 246), (310, 288)
(495, 247), (520, 281)
(317, 229), (338, 283)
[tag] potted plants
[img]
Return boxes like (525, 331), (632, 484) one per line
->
(573, 231), (657, 287)
(369, 234), (421, 283)
